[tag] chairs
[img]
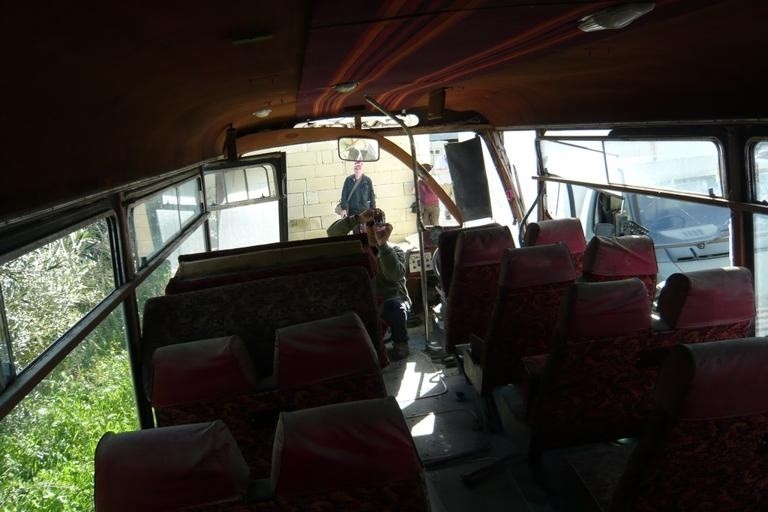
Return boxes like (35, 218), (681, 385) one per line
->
(153, 314), (386, 465)
(93, 397), (429, 512)
(495, 337), (767, 511)
(143, 232), (389, 404)
(436, 219), (758, 417)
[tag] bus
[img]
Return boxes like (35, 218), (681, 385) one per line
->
(546, 170), (768, 335)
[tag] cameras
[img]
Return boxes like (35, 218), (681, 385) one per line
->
(366, 210), (386, 231)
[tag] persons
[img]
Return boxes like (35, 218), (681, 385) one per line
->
(339, 160), (377, 234)
(432, 144), (457, 220)
(416, 162), (439, 227)
(325, 206), (414, 361)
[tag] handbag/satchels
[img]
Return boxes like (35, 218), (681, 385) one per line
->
(334, 202), (349, 219)
